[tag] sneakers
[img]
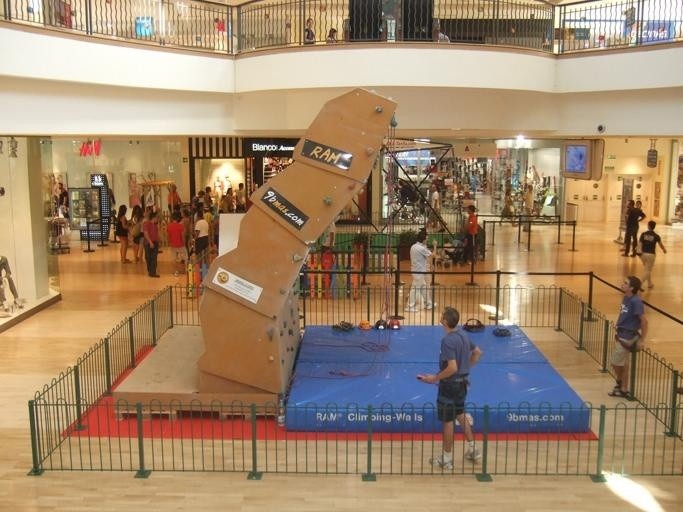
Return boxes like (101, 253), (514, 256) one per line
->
(405, 304), (419, 313)
(424, 301), (439, 310)
(429, 457), (453, 471)
(647, 282), (655, 288)
(464, 449), (481, 460)
(630, 254), (636, 257)
(149, 274), (160, 278)
(621, 253), (628, 257)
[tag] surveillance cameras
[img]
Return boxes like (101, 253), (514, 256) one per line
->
(598, 125), (606, 133)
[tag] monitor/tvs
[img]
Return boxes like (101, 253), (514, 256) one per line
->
(590, 139), (605, 181)
(560, 139), (592, 179)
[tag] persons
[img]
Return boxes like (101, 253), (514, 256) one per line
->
(419, 306), (483, 470)
(619, 200), (643, 252)
(0, 255), (22, 312)
(607, 275), (649, 398)
(304, 17), (316, 44)
(638, 220), (668, 292)
(403, 230), (438, 312)
(432, 25), (450, 43)
(115, 182), (245, 279)
(58, 182), (70, 218)
(320, 221), (335, 258)
(570, 146), (585, 171)
(621, 199), (646, 258)
(325, 28), (337, 43)
(41, 175), (63, 205)
(418, 171), (541, 266)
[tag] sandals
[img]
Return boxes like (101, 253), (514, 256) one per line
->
(613, 379), (622, 391)
(607, 388), (629, 398)
